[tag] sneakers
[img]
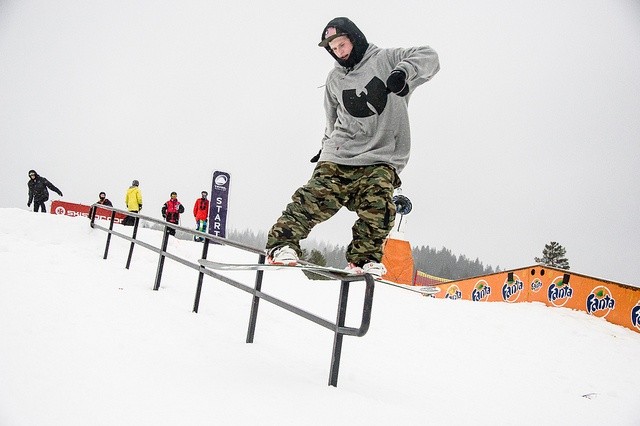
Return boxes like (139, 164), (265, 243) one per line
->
(268, 247), (298, 266)
(346, 262), (388, 277)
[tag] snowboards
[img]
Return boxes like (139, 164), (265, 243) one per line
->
(198, 259), (440, 298)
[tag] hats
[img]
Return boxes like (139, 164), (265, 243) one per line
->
(319, 25), (350, 46)
(29, 171), (35, 176)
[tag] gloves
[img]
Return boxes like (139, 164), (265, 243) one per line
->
(28, 203), (30, 207)
(384, 69), (409, 97)
(311, 151), (321, 163)
(59, 192), (63, 195)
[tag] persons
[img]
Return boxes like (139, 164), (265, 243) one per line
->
(161, 192), (184, 236)
(93, 192), (113, 208)
(264, 16), (441, 277)
(27, 170), (62, 212)
(193, 191), (209, 243)
(122, 180), (143, 225)
